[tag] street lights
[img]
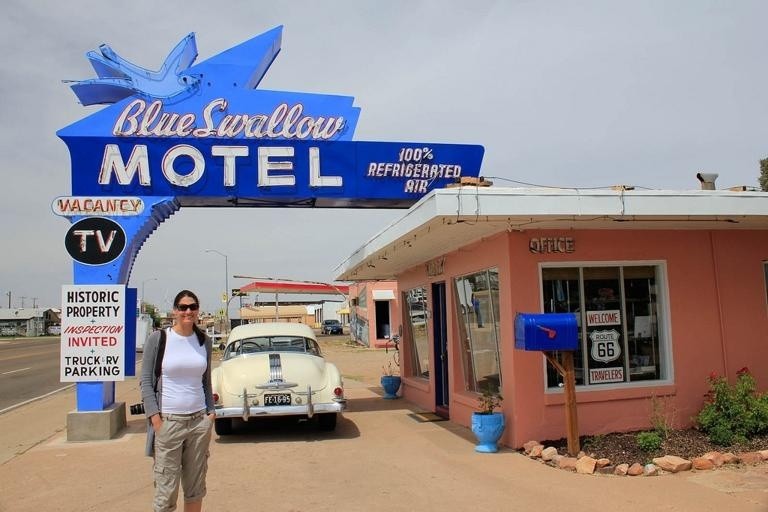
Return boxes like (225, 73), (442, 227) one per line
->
(205, 249), (228, 321)
(141, 278), (157, 302)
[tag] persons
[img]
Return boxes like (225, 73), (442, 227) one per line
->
(140, 290), (216, 512)
(471, 293), (485, 328)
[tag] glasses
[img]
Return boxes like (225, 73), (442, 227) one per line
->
(176, 304), (198, 312)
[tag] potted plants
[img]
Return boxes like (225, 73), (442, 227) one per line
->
(471, 380), (506, 456)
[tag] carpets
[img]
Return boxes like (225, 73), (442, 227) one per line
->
(406, 412), (448, 422)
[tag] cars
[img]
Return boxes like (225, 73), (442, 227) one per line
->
(320, 320), (343, 335)
(210, 323), (346, 434)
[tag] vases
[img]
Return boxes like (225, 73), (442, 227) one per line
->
(381, 375), (401, 399)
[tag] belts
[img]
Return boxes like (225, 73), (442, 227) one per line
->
(162, 410), (207, 421)
